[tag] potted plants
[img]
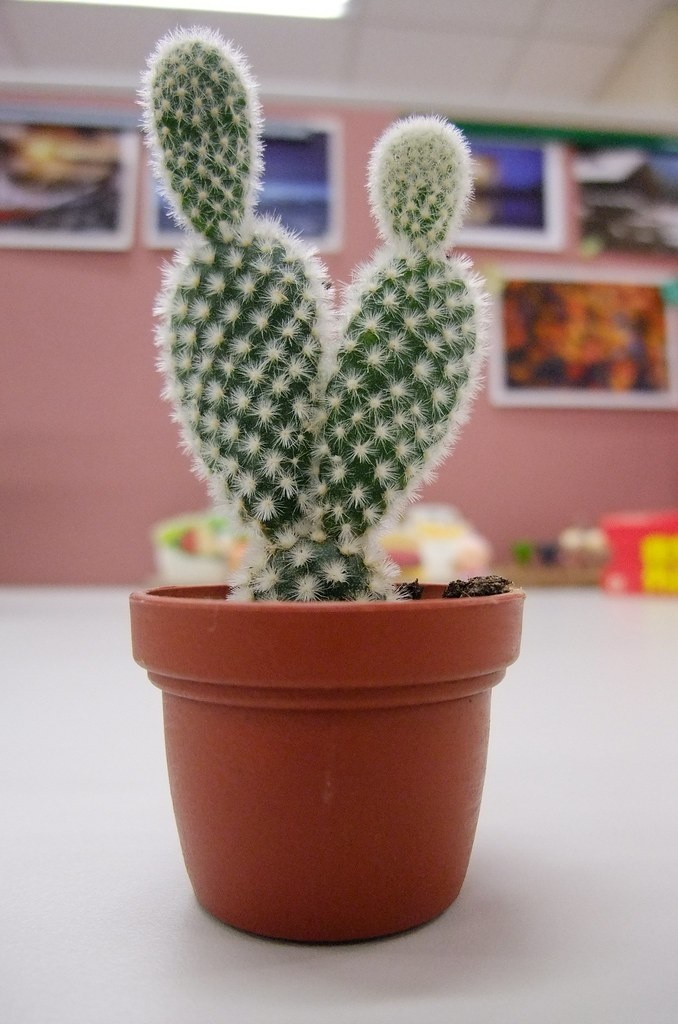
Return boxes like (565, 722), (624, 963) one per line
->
(125, 24), (527, 944)
